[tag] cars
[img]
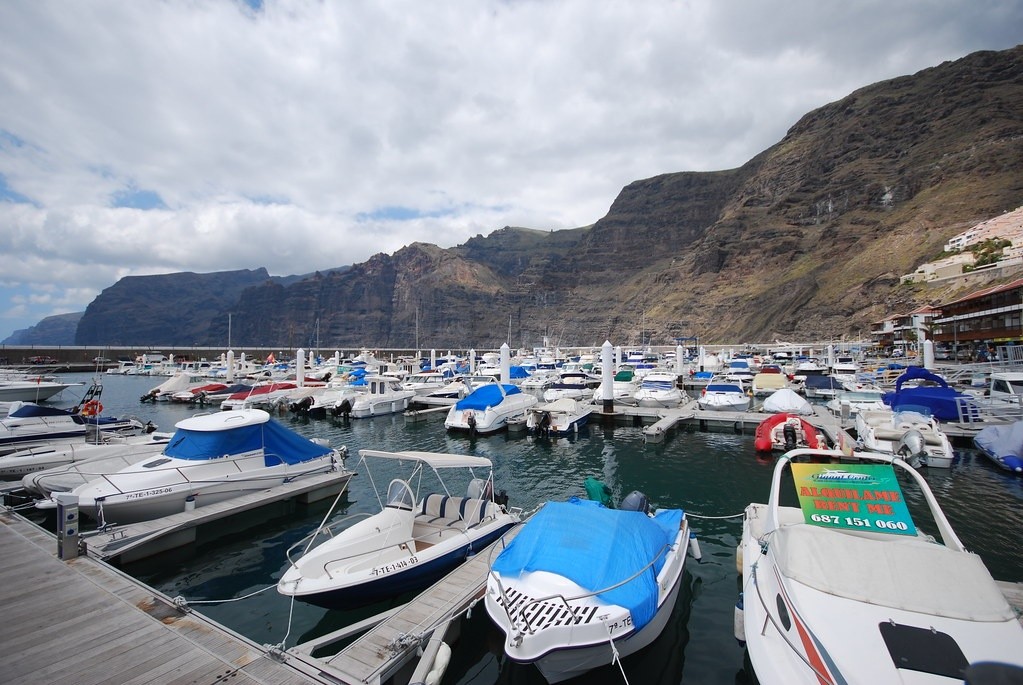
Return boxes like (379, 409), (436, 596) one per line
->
(31, 356), (59, 364)
(92, 357), (112, 364)
(663, 351), (676, 358)
(246, 355), (254, 360)
(213, 355), (227, 360)
(865, 346), (1005, 361)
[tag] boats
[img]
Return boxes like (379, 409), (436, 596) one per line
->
(486, 489), (692, 683)
(277, 448), (527, 613)
(974, 419), (1023, 474)
(527, 395), (591, 436)
(854, 407), (954, 470)
(732, 449), (1022, 685)
(34, 401), (350, 523)
(757, 412), (828, 455)
(963, 371), (1023, 416)
(0, 368), (170, 500)
(105, 346), (978, 419)
(446, 376), (536, 436)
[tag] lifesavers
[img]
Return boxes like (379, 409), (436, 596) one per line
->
(84, 400), (103, 415)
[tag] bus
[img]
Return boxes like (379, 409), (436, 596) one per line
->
(173, 354), (194, 362)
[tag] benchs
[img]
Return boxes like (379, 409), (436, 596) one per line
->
(413, 492), (493, 535)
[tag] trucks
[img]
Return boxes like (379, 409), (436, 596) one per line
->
(142, 354), (168, 363)
